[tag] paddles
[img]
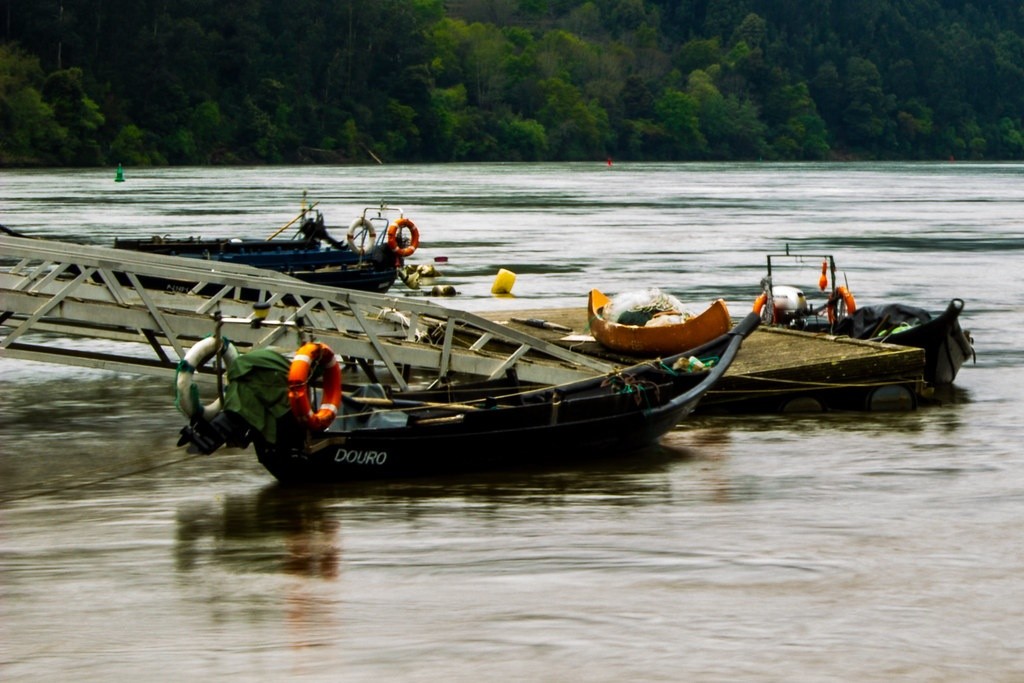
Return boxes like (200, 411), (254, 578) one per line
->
(266, 200), (320, 241)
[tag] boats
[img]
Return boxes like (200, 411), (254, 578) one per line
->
(251, 313), (761, 486)
(766, 243), (973, 388)
(587, 288), (733, 357)
(0, 192), (404, 306)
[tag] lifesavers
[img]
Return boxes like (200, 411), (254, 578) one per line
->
(177, 336), (241, 421)
(288, 341), (342, 432)
(347, 217), (376, 253)
(754, 293), (777, 326)
(826, 286), (856, 325)
(386, 218), (420, 257)
